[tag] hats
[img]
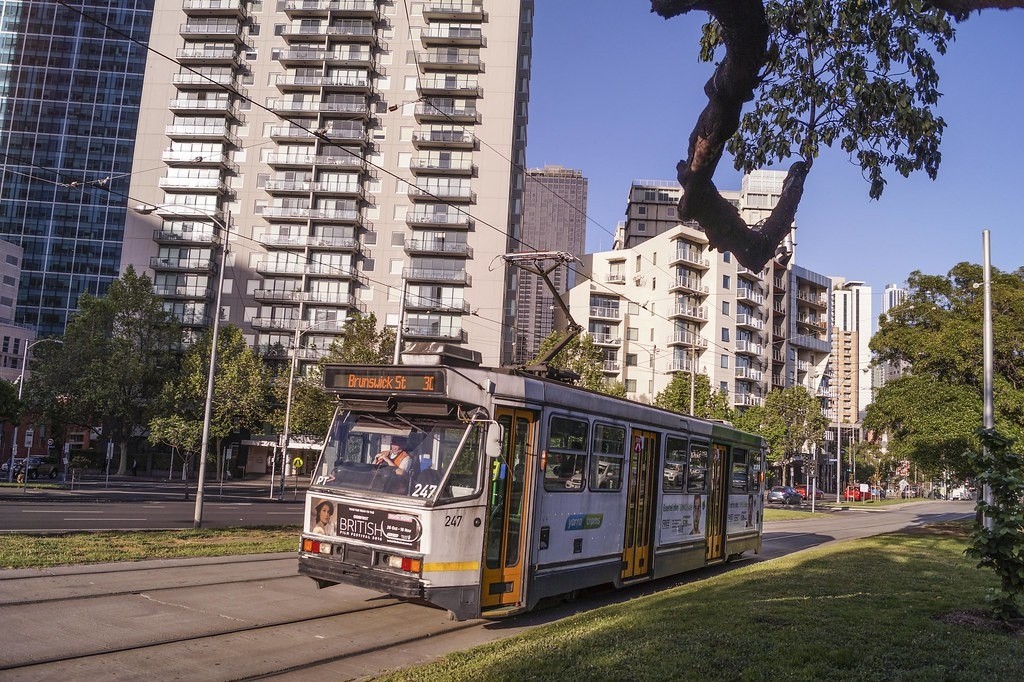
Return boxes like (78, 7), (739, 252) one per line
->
(391, 436), (407, 445)
(315, 500), (334, 516)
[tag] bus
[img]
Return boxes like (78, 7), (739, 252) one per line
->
(297, 342), (766, 622)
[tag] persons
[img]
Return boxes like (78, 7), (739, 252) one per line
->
(512, 464), (525, 492)
(372, 435), (411, 495)
(553, 441), (584, 478)
(313, 499), (334, 535)
(746, 495), (754, 527)
(689, 495), (702, 535)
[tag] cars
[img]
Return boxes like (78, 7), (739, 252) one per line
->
(663, 463), (684, 486)
(2, 458), (24, 472)
(844, 486), (873, 502)
(870, 486), (886, 499)
(566, 461), (637, 497)
(693, 468), (706, 481)
(767, 487), (804, 506)
(794, 485), (825, 501)
(946, 489), (972, 501)
(928, 492), (945, 500)
(901, 487), (926, 498)
(732, 471), (753, 490)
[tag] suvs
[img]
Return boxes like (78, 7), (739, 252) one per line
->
(13, 455), (60, 481)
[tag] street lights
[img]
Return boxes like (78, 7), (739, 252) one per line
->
(278, 317), (356, 499)
(8, 338), (64, 482)
(134, 202), (233, 530)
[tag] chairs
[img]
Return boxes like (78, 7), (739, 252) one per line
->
(391, 450), (420, 494)
